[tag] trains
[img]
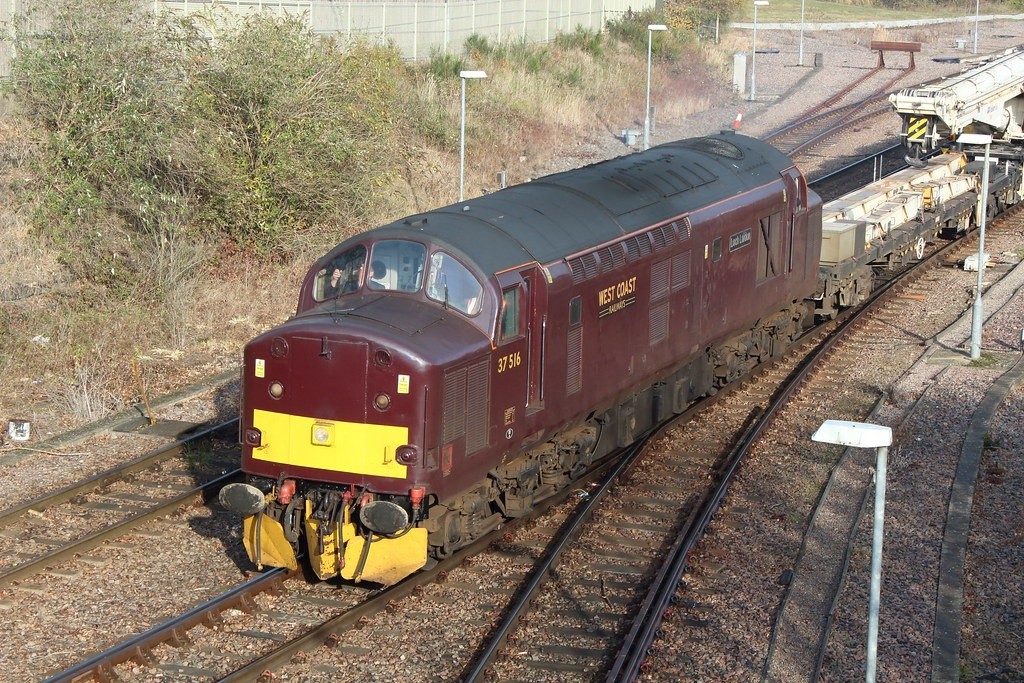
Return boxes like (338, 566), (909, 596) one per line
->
(219, 45), (1024, 589)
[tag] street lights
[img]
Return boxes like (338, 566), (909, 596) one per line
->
(749, 0), (769, 101)
(956, 130), (994, 359)
(458, 69), (489, 202)
(643, 24), (669, 151)
(812, 419), (894, 683)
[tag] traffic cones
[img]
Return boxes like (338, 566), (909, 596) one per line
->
(731, 107), (745, 131)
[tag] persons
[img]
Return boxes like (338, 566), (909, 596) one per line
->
(329, 260), (383, 296)
